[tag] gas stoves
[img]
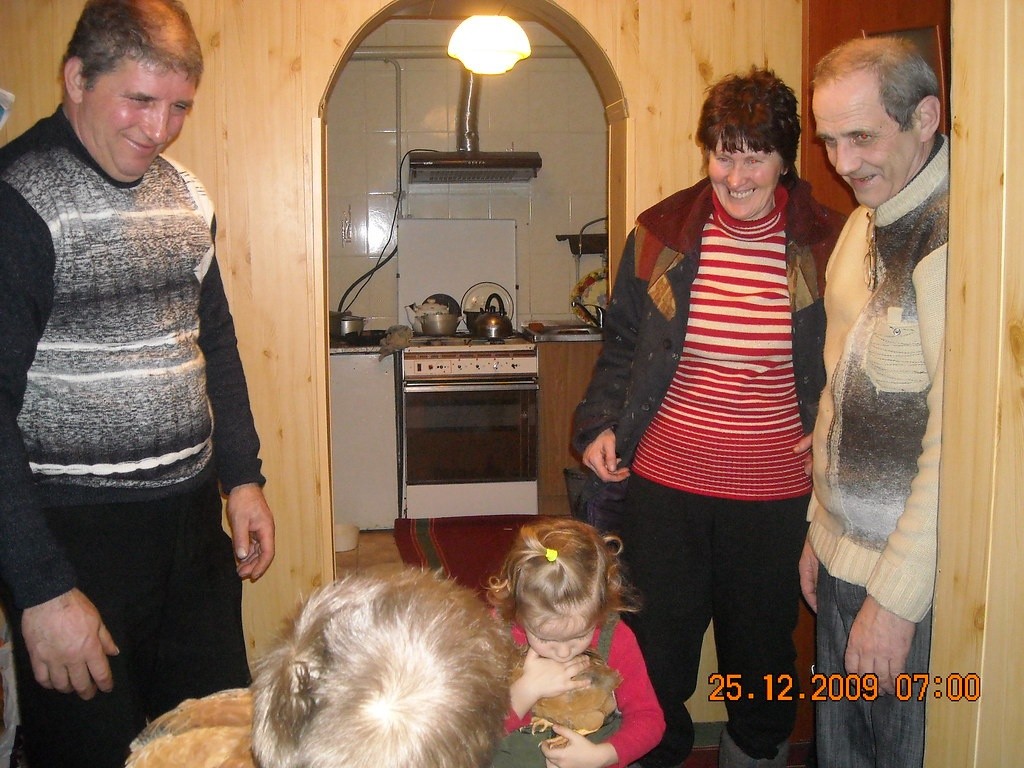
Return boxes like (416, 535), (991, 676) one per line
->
(402, 333), (540, 383)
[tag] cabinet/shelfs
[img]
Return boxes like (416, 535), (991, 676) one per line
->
(535, 342), (603, 514)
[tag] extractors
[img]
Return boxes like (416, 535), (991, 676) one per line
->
(407, 61), (542, 183)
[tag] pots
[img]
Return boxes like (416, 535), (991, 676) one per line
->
(330, 311), (342, 336)
(344, 329), (391, 346)
(341, 311), (366, 338)
(405, 298), (450, 335)
(556, 233), (607, 255)
(464, 309), (501, 334)
(415, 311), (463, 337)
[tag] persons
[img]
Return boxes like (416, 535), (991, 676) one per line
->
(798, 35), (952, 768)
(483, 517), (666, 767)
(124, 562), (529, 768)
(573, 64), (847, 768)
(0, 0), (276, 768)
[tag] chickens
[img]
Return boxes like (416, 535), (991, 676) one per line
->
(124, 687), (259, 768)
(510, 644), (627, 751)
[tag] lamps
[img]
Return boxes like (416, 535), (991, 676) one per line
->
(448, 15), (532, 74)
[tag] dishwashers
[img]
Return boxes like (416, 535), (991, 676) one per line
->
(400, 382), (540, 517)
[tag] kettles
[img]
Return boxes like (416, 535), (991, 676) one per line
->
(474, 293), (514, 338)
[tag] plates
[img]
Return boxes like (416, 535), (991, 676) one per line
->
(422, 294), (462, 329)
(460, 281), (513, 324)
